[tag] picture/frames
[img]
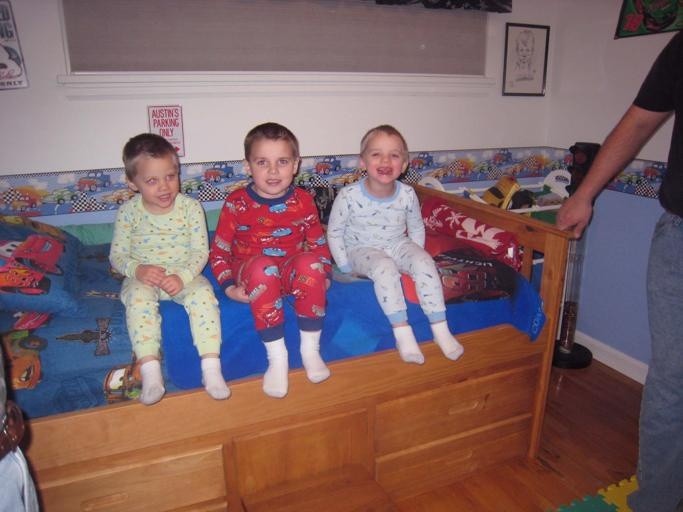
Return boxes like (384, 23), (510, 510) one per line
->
(501, 21), (551, 96)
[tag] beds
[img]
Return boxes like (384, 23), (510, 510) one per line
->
(0, 182), (573, 512)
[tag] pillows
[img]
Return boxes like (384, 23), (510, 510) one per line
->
(402, 198), (525, 305)
(0, 220), (89, 320)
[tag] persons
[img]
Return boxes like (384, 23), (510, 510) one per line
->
(105, 131), (233, 406)
(556, 30), (682, 510)
(209, 121), (334, 396)
(513, 29), (537, 83)
(324, 124), (465, 365)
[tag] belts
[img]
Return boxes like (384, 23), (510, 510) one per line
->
(0, 402), (24, 461)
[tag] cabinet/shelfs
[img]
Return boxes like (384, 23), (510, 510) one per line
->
(419, 170), (571, 340)
